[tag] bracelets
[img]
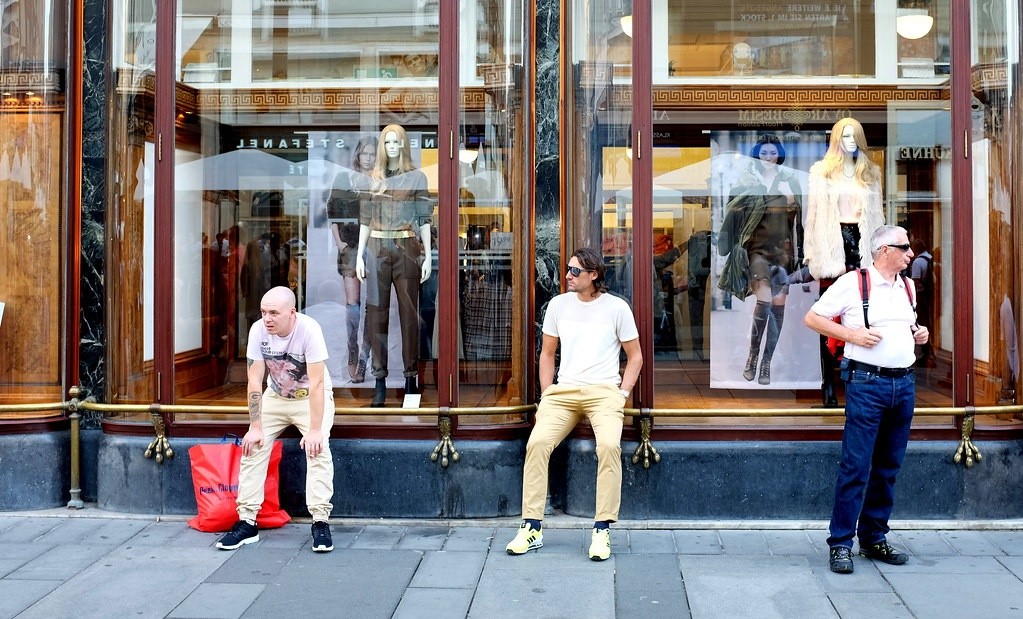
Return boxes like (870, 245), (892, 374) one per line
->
(620, 390), (629, 399)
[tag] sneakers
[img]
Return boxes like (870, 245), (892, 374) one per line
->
(859, 540), (909, 565)
(589, 526), (612, 561)
(311, 520), (334, 551)
(506, 522), (544, 555)
(829, 545), (855, 574)
(216, 519), (260, 550)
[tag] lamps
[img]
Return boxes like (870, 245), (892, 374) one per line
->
(895, 1), (934, 41)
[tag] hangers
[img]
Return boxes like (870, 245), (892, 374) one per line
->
(476, 266), (502, 283)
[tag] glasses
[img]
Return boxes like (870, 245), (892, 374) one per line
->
(565, 263), (597, 277)
(877, 243), (913, 251)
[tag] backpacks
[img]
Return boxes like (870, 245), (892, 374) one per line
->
(917, 256), (935, 294)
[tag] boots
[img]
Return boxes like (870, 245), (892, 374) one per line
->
(346, 303), (361, 377)
(758, 304), (785, 385)
(404, 376), (418, 394)
(371, 379), (387, 407)
(743, 300), (772, 381)
(352, 307), (371, 383)
(820, 335), (839, 409)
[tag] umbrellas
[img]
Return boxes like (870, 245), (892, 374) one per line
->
(152, 149), (854, 215)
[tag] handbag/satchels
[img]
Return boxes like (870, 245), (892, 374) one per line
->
(188, 434), (291, 532)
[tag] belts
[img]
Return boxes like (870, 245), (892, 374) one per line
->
(849, 359), (915, 378)
(369, 229), (416, 239)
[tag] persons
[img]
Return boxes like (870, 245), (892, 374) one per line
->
(716, 135), (806, 385)
(324, 136), (380, 384)
(909, 236), (939, 367)
(803, 115), (886, 407)
(803, 226), (931, 575)
(196, 225), (307, 338)
(402, 54), (433, 77)
(350, 125), (433, 409)
(217, 286), (337, 552)
(505, 248), (646, 560)
(620, 234), (670, 322)
(993, 292), (1020, 391)
